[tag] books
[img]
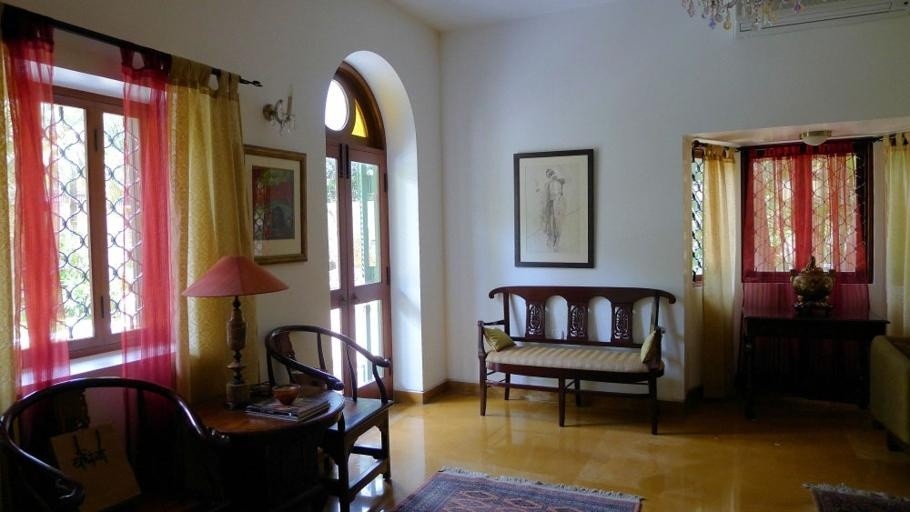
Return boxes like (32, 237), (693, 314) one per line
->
(249, 394), (330, 415)
(244, 400), (333, 421)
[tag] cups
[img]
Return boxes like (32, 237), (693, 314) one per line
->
(273, 383), (302, 405)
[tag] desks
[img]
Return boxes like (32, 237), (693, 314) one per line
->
(192, 384), (347, 512)
(743, 311), (890, 407)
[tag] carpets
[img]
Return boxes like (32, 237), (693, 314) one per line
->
(391, 466), (645, 512)
(803, 484), (910, 512)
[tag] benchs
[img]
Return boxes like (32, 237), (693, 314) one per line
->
(478, 286), (677, 435)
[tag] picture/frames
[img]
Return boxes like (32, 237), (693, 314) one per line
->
(243, 143), (309, 265)
(513, 149), (594, 268)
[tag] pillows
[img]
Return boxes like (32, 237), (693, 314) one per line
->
(484, 328), (516, 352)
(641, 331), (657, 362)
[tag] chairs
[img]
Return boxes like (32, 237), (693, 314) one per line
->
(868, 336), (910, 450)
(264, 325), (395, 512)
(0, 376), (232, 512)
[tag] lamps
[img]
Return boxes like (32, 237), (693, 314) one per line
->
(799, 130), (833, 145)
(180, 253), (290, 410)
(263, 86), (299, 134)
